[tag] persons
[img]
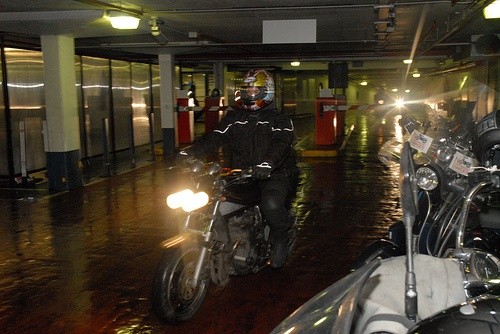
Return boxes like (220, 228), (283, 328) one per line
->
(187, 85), (199, 123)
(184, 69), (301, 270)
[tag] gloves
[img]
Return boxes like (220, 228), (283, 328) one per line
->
(175, 150), (195, 166)
(252, 162), (274, 182)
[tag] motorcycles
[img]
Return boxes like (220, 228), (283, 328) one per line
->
(151, 149), (302, 323)
(269, 143), (499, 334)
(348, 103), (499, 275)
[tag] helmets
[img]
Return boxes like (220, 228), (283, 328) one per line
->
(235, 69), (274, 111)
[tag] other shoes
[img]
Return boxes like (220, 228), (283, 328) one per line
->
(270, 229), (289, 270)
